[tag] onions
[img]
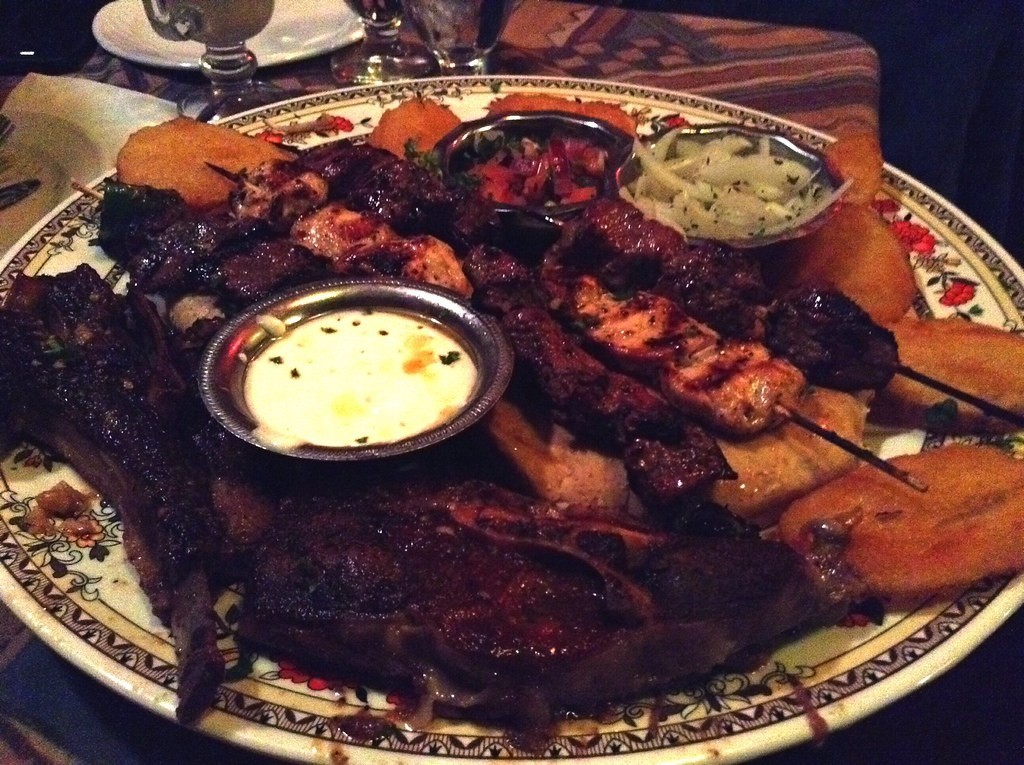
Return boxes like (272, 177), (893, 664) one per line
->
(513, 135), (604, 194)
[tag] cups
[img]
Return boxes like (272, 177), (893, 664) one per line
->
(410, 1), (511, 76)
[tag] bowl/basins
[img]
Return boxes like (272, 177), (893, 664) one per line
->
(430, 110), (626, 221)
(199, 278), (511, 464)
(611, 124), (848, 251)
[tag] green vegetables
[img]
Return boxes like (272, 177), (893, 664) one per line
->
(400, 133), (503, 187)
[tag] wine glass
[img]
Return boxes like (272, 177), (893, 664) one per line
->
(143, 1), (288, 120)
(334, 0), (436, 88)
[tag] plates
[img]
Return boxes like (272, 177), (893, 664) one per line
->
(0, 77), (1024, 765)
(93, 1), (366, 70)
